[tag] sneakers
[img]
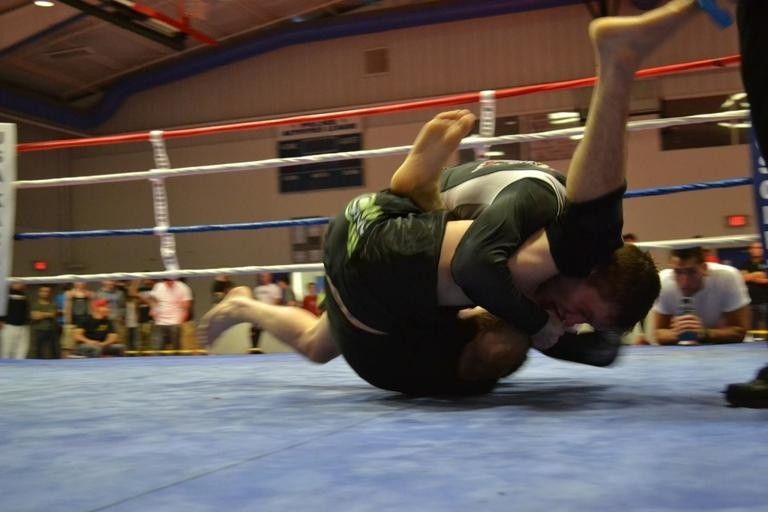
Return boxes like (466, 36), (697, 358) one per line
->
(726, 363), (768, 409)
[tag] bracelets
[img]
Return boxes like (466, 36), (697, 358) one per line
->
(701, 324), (713, 344)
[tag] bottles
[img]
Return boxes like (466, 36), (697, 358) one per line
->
(674, 295), (700, 346)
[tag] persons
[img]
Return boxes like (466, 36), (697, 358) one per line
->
(318, 2), (712, 405)
(640, 237), (754, 347)
(736, 238), (767, 340)
(621, 233), (647, 346)
(714, 2), (768, 409)
(211, 270), (318, 355)
(2, 271), (192, 360)
(192, 154), (664, 369)
